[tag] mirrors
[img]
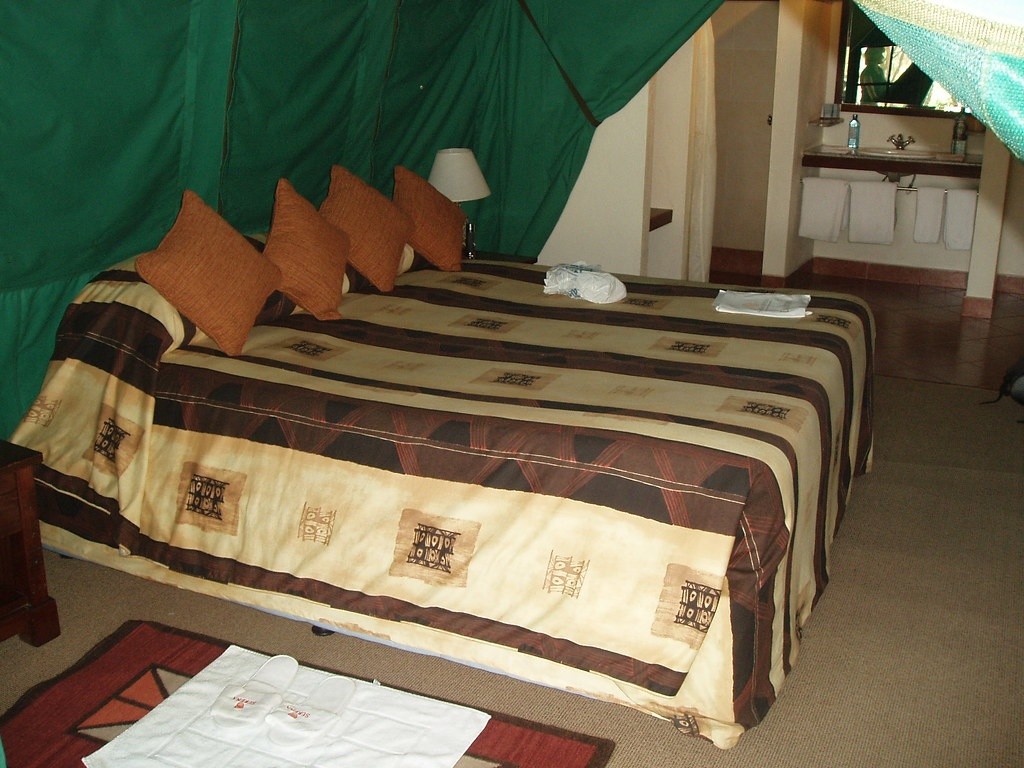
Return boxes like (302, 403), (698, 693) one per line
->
(834, 0), (977, 121)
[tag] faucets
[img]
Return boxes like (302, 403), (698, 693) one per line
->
(897, 133), (904, 150)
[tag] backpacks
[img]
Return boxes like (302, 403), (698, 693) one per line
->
(979, 351), (1024, 424)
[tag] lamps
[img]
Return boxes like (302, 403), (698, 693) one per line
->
(428, 148), (491, 258)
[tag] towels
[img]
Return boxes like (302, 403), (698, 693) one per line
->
(80, 645), (492, 768)
(913, 187), (945, 244)
(943, 188), (977, 251)
(798, 176), (850, 243)
(849, 181), (897, 245)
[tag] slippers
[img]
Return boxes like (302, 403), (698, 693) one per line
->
(211, 655), (356, 746)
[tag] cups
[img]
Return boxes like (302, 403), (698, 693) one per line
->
(833, 104), (841, 118)
(821, 103), (832, 117)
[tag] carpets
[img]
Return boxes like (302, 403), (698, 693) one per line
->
(81, 645), (492, 768)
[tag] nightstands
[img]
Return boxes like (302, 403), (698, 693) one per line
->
(0, 439), (61, 647)
(486, 252), (537, 264)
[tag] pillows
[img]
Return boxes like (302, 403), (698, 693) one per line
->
(263, 179), (351, 320)
(318, 165), (417, 292)
(135, 190), (283, 355)
(392, 165), (467, 271)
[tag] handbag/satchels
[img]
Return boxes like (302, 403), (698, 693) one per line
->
(543, 263), (627, 303)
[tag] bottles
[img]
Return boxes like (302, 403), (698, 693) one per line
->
(952, 107), (968, 154)
(848, 114), (860, 148)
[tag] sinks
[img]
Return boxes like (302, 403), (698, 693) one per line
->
(856, 147), (940, 159)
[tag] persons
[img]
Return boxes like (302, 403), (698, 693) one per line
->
(860, 47), (888, 102)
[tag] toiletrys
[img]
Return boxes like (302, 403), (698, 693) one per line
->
(847, 114), (861, 150)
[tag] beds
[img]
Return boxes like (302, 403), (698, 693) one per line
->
(12, 233), (875, 747)
